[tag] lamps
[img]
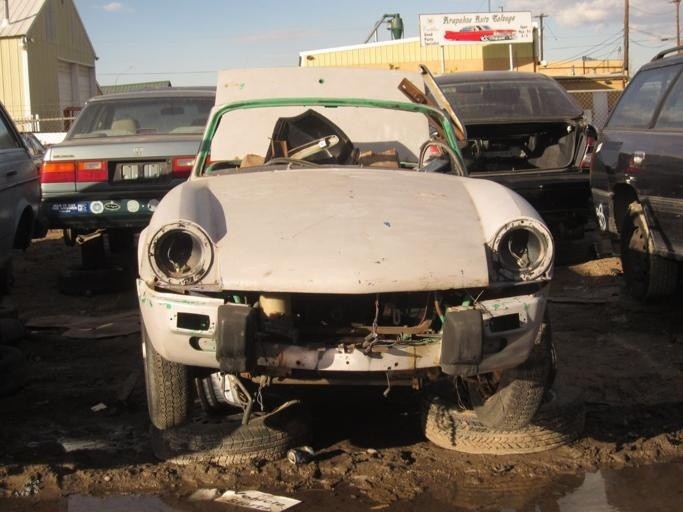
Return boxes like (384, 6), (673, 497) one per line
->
(239, 153), (267, 168)
(360, 148), (401, 172)
(191, 117), (205, 127)
(111, 119), (139, 136)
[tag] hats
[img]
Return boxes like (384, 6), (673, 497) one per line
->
(443, 24), (517, 42)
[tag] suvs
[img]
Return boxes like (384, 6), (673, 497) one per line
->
(590, 46), (682, 305)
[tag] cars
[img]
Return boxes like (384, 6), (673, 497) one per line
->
(431, 70), (603, 240)
(135, 67), (556, 430)
(19, 131), (45, 169)
(443, 24), (517, 42)
(1, 103), (42, 290)
(41, 90), (217, 240)
